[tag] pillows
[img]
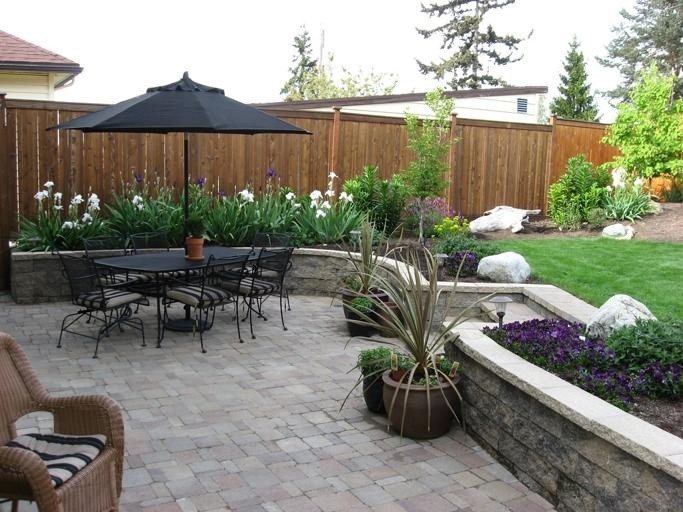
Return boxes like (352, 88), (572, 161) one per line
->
(4, 432), (108, 488)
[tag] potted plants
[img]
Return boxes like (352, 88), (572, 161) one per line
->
(184, 219), (207, 256)
(329, 244), (498, 440)
(335, 208), (411, 337)
(357, 347), (412, 415)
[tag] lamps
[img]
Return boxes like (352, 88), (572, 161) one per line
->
(433, 253), (449, 281)
(489, 296), (513, 327)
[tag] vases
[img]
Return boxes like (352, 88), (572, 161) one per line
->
(376, 302), (404, 337)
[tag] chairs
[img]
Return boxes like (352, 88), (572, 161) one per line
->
(0, 331), (124, 512)
(47, 232), (303, 358)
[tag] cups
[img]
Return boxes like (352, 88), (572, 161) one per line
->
(186, 238), (204, 257)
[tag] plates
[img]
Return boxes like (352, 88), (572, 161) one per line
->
(184, 255), (205, 260)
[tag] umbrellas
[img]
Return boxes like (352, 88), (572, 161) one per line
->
(42, 70), (315, 256)
